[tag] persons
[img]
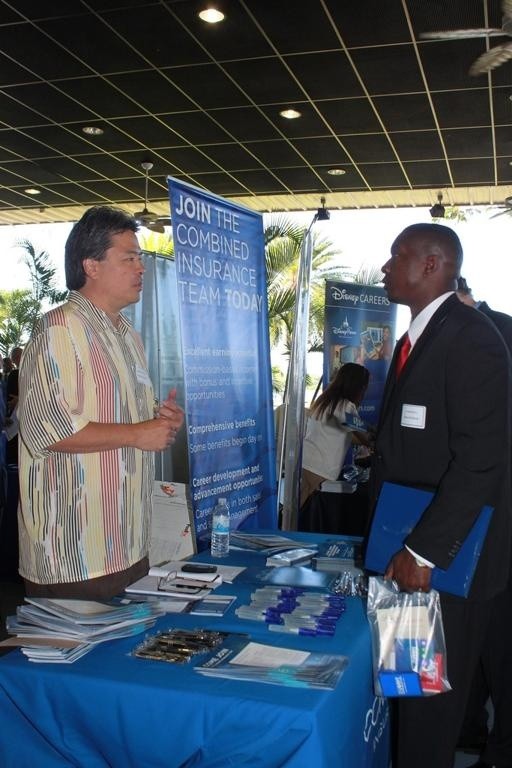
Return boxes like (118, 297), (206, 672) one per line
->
(456, 275), (512, 361)
(375, 325), (392, 361)
(353, 223), (512, 768)
(19, 204), (185, 606)
(297, 362), (375, 509)
(0, 347), (23, 471)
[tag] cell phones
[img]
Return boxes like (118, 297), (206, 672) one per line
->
(182, 565), (217, 572)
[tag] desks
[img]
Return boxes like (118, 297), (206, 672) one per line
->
(1, 531), (381, 767)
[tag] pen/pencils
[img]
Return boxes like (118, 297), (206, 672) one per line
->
(170, 584), (214, 591)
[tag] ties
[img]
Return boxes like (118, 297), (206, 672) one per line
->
(395, 335), (411, 382)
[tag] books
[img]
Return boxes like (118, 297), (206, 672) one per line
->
(125, 560), (247, 601)
(364, 482), (495, 599)
(0, 598), (166, 664)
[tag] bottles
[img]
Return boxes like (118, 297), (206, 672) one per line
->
(211, 499), (233, 560)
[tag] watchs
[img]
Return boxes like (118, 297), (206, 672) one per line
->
(415, 558), (427, 567)
